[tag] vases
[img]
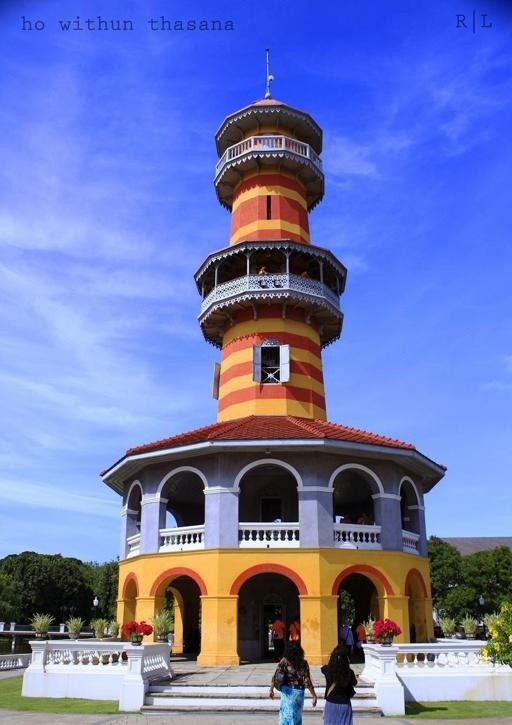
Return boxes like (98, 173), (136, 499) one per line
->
(379, 630), (393, 646)
(131, 635), (143, 646)
(434, 626), (440, 638)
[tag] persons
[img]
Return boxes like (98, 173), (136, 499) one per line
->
(288, 619), (300, 642)
(271, 619), (286, 661)
(268, 640), (317, 725)
(354, 621), (366, 649)
(320, 644), (358, 725)
(339, 619), (354, 663)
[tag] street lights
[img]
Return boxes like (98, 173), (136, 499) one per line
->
(92, 595), (98, 637)
(480, 594), (487, 640)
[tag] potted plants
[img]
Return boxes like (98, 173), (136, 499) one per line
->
(358, 621), (375, 641)
(26, 612), (57, 638)
(90, 616), (110, 638)
(439, 617), (458, 639)
(64, 615), (86, 640)
(153, 609), (174, 643)
(109, 620), (119, 637)
(459, 612), (477, 640)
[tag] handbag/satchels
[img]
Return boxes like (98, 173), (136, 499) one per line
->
(275, 670), (287, 684)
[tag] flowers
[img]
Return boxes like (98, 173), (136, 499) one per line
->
(121, 620), (153, 640)
(373, 619), (402, 644)
